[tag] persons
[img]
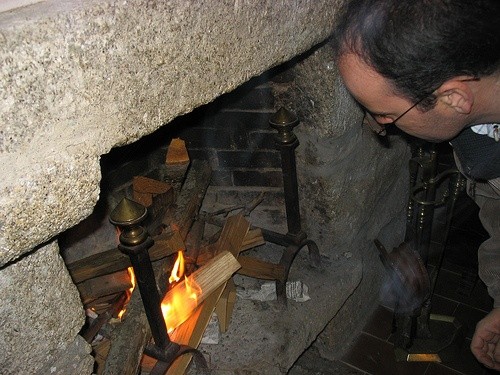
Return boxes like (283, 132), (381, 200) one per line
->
(331, 0), (500, 370)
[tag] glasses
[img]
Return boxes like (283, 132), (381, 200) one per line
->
(362, 70), (480, 136)
